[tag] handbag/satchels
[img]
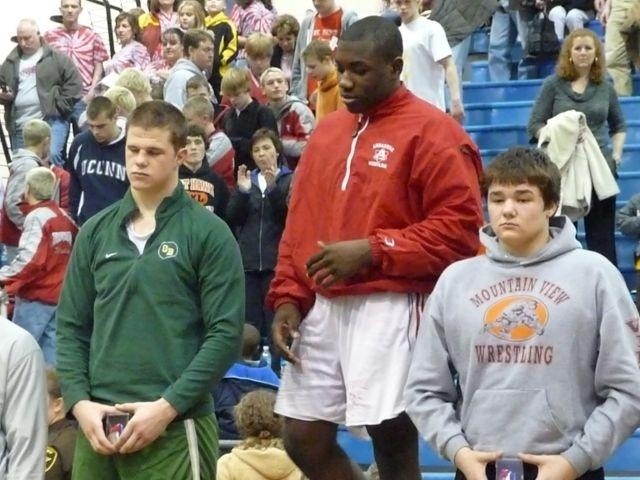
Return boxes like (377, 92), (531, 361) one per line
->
(528, 12), (560, 54)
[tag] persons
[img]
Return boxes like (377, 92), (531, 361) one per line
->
(56, 101), (247, 480)
(265, 17), (487, 480)
(486, 0), (640, 99)
(45, 368), (77, 480)
(214, 389), (306, 480)
(211, 324), (281, 439)
(403, 148), (640, 480)
(615, 192), (640, 315)
(0, 0), (470, 366)
(527, 29), (628, 270)
(0, 316), (48, 480)
